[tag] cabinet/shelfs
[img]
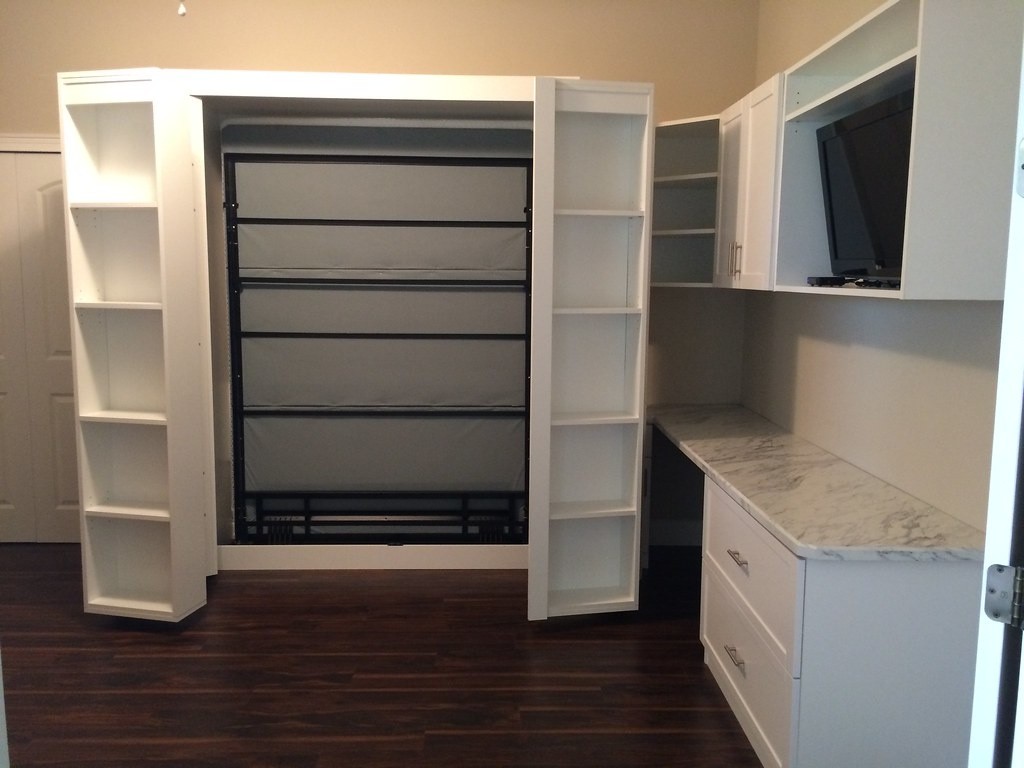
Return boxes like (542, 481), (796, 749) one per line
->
(700, 473), (983, 768)
(649, 114), (720, 287)
(57, 68), (655, 623)
(717, 71), (785, 290)
(774, 1), (1023, 300)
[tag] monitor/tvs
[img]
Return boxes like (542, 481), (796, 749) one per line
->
(815, 86), (916, 280)
